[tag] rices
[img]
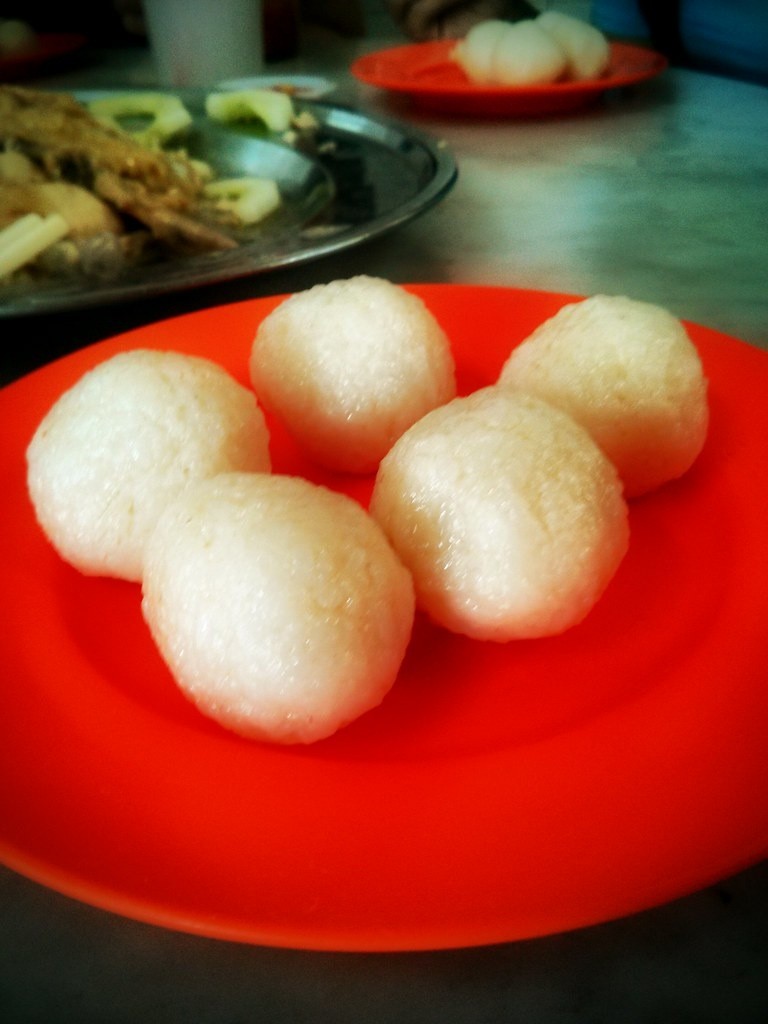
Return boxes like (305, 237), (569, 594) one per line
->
(28, 277), (707, 747)
(450, 8), (610, 85)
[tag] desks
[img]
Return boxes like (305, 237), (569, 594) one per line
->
(0, 49), (768, 1024)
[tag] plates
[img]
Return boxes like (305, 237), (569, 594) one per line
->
(0, 85), (459, 341)
(1, 280), (767, 951)
(212, 72), (338, 107)
(349, 41), (666, 118)
